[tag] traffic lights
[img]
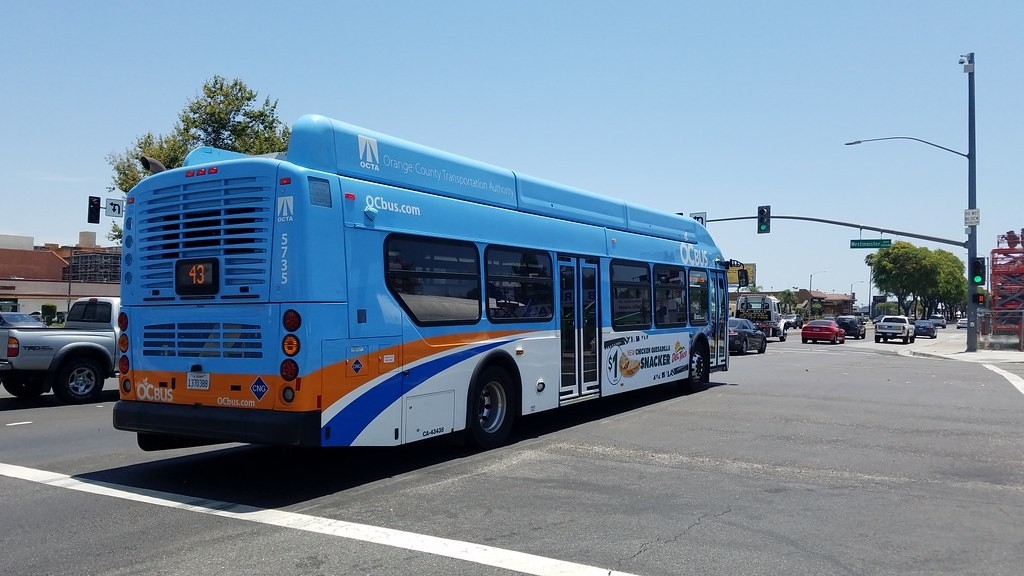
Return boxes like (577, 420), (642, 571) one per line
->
(88, 196), (100, 224)
(758, 206), (770, 234)
(973, 293), (984, 303)
(971, 257), (986, 285)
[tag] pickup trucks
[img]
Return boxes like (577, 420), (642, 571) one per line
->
(874, 315), (916, 344)
(0, 297), (120, 405)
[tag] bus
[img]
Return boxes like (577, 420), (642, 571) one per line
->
(112, 114), (750, 462)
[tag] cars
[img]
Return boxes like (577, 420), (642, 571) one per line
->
(823, 317), (836, 322)
(801, 319), (846, 344)
(729, 318), (766, 355)
(859, 315), (885, 325)
(914, 320), (938, 339)
(0, 312), (49, 330)
(957, 319), (968, 329)
(28, 311), (68, 324)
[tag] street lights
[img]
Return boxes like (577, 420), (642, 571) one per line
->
(808, 270), (827, 322)
(844, 136), (979, 353)
(850, 280), (864, 315)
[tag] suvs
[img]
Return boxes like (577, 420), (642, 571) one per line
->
(784, 314), (803, 329)
(836, 315), (866, 340)
(929, 315), (946, 329)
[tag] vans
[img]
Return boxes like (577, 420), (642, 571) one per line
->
(736, 294), (788, 341)
